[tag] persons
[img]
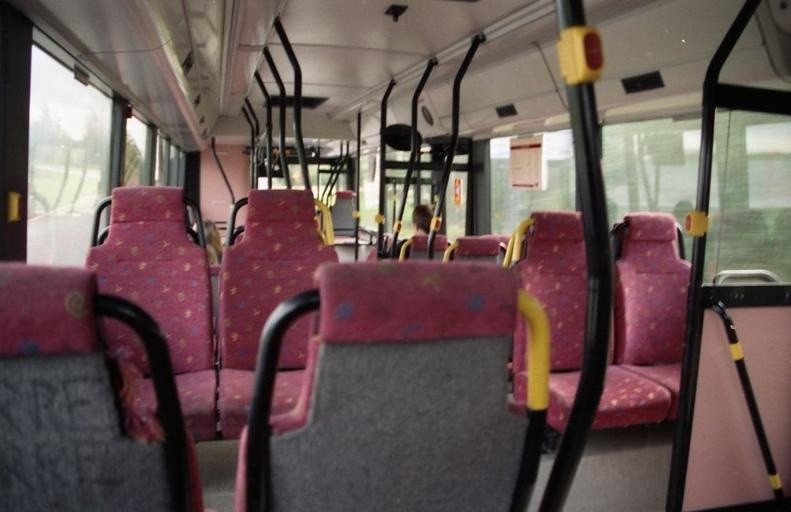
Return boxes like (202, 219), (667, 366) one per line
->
(396, 204), (433, 258)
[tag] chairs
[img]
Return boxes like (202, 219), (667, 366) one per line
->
(1, 262), (205, 512)
(331, 190), (357, 236)
(82, 184), (218, 444)
(233, 260), (551, 512)
(507, 209), (672, 434)
(613, 212), (692, 420)
(217, 190), (339, 440)
(441, 236), (506, 265)
(397, 234), (451, 263)
